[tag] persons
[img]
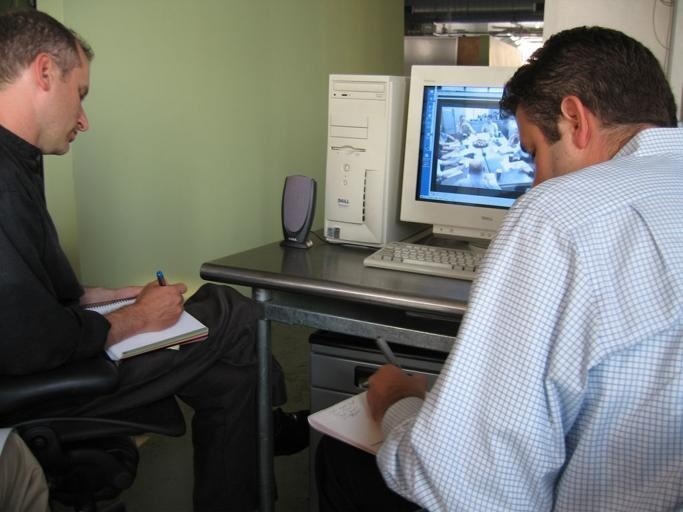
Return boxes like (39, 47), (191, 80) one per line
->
(307, 28), (682, 510)
(0, 8), (308, 509)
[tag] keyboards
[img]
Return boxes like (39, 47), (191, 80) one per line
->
(364, 241), (485, 281)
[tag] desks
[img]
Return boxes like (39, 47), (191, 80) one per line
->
(197, 228), (485, 512)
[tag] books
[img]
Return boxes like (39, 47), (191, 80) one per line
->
(83, 291), (213, 364)
(306, 381), (430, 458)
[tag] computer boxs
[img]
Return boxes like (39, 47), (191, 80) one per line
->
(323, 74), (433, 248)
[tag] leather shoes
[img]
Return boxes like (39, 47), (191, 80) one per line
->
(272, 407), (310, 457)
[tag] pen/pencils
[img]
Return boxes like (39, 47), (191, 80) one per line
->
(375, 335), (401, 369)
(156, 271), (167, 286)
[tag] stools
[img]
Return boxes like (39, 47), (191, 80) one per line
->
(1, 379), (189, 510)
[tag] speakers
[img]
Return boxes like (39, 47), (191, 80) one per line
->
(280, 175), (317, 249)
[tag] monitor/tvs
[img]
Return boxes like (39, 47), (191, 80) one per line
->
(400, 65), (537, 254)
